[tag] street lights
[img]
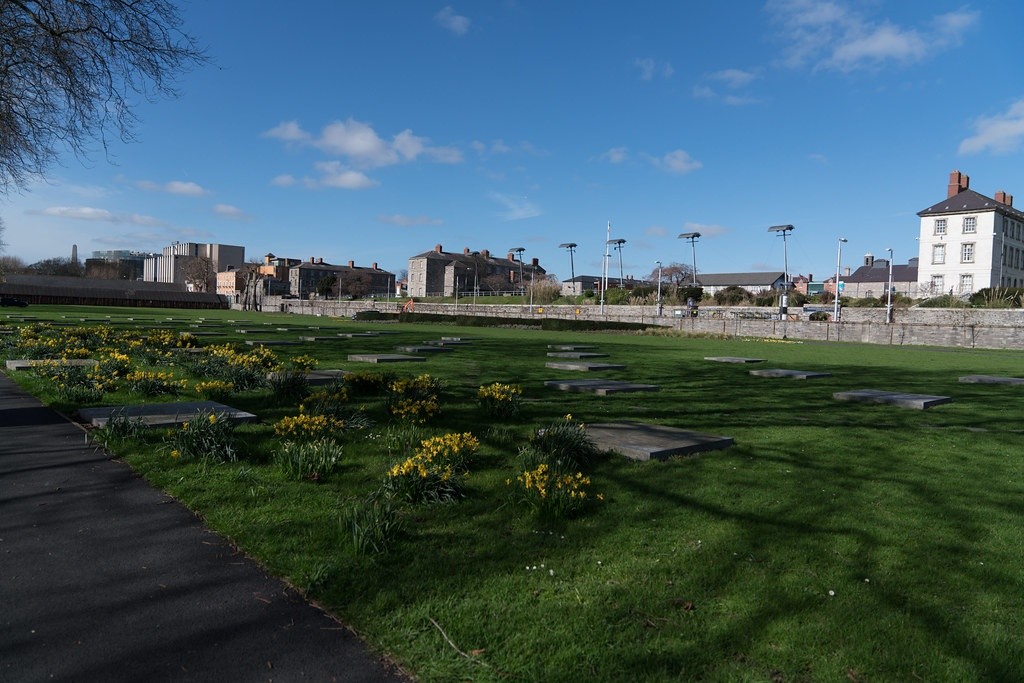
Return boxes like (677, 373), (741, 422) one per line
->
(507, 247), (525, 297)
(678, 231), (701, 286)
(652, 260), (662, 316)
(833, 237), (848, 323)
(604, 240), (628, 290)
(886, 247), (894, 324)
(768, 225), (795, 321)
(464, 251), (481, 296)
(559, 242), (577, 294)
